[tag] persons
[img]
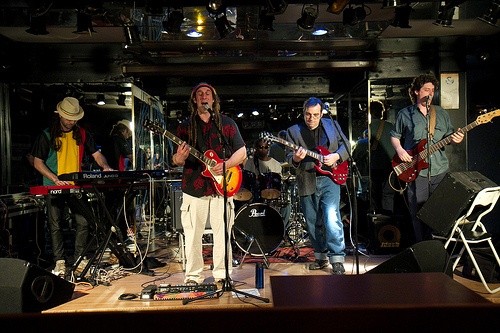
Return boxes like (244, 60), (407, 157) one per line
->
(243, 98), (352, 275)
(389, 74), (464, 243)
(352, 101), (395, 210)
(29, 97), (119, 274)
(172, 82), (247, 287)
(92, 120), (137, 237)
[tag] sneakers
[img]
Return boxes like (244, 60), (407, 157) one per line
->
(51, 260), (66, 276)
(309, 259), (329, 270)
(332, 262), (345, 272)
(74, 259), (98, 277)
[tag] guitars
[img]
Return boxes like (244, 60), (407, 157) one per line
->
(141, 117), (243, 197)
(261, 132), (350, 185)
(390, 105), (499, 185)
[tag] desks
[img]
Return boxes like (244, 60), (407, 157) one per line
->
(270, 272), (494, 308)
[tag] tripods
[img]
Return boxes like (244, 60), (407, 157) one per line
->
(180, 119), (270, 305)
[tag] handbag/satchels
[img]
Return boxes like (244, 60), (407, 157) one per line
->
(462, 247), (500, 284)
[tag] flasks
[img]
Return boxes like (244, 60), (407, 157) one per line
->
(255, 262), (264, 289)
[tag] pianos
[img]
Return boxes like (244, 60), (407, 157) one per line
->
(58, 169), (164, 286)
(30, 185), (156, 287)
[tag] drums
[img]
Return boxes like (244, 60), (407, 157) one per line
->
(259, 172), (282, 200)
(229, 170), (255, 201)
(230, 201), (285, 257)
(281, 164), (296, 181)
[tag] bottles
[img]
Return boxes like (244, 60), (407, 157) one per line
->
(135, 204), (140, 217)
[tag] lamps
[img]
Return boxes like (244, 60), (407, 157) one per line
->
(326, 0), (372, 26)
(381, 0), (420, 29)
(161, 4), (186, 34)
(24, 0), (49, 35)
(96, 93), (105, 105)
(115, 94), (126, 106)
(432, 0), (465, 28)
(71, 7), (98, 34)
(476, 0), (500, 28)
(204, 0), (288, 42)
(230, 103), (302, 123)
(296, 0), (319, 32)
(121, 23), (143, 49)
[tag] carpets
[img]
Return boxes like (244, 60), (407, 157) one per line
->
(202, 245), (314, 264)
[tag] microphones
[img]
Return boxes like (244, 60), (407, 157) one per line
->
(204, 104), (215, 118)
(323, 102), (330, 111)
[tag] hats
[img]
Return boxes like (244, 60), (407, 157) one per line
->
(190, 83), (217, 102)
(57, 97), (85, 121)
(278, 130), (287, 135)
(117, 119), (132, 137)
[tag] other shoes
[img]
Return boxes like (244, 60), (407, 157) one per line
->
(186, 279), (197, 286)
(214, 278), (223, 282)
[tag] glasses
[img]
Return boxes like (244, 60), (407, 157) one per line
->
(146, 152), (150, 154)
(156, 146), (160, 147)
(257, 145), (270, 149)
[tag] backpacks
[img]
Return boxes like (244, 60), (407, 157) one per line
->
(283, 212), (313, 248)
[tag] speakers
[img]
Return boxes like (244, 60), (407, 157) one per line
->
(415, 170), (499, 238)
(0, 258), (75, 314)
(363, 238), (455, 279)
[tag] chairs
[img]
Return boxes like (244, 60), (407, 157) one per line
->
(431, 186), (500, 293)
